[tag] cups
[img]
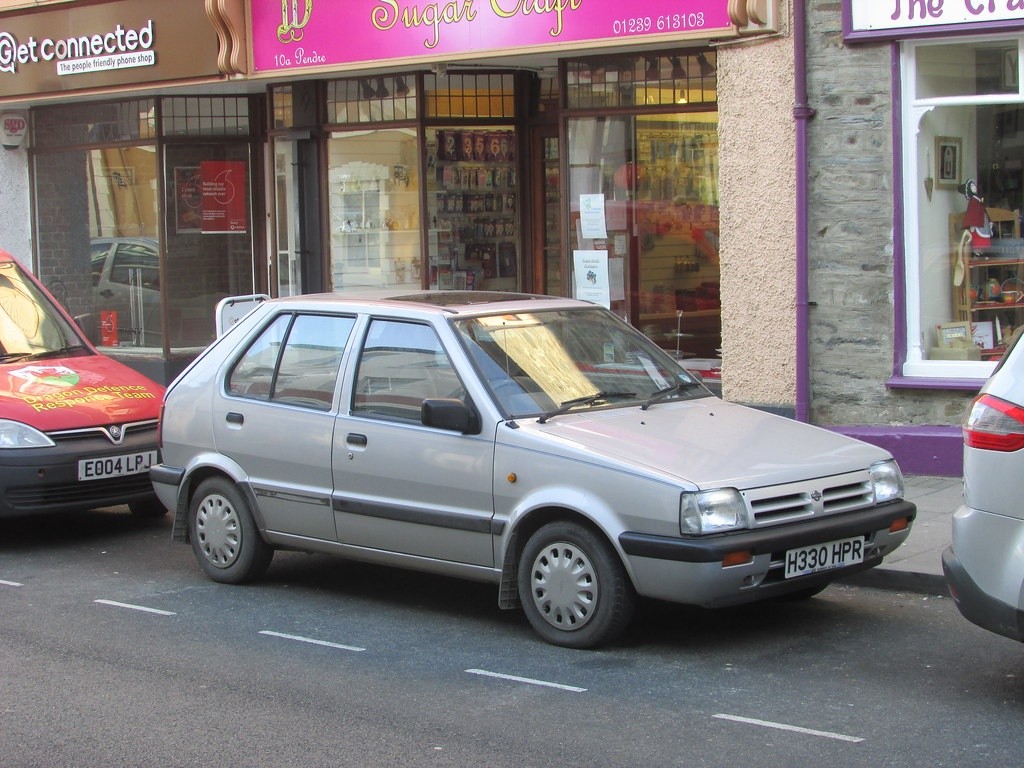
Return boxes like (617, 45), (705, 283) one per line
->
(1001, 290), (1022, 304)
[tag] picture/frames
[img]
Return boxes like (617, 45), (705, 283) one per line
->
(936, 320), (972, 348)
(934, 136), (963, 189)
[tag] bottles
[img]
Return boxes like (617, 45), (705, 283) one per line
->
(982, 278), (1002, 302)
(962, 283), (978, 309)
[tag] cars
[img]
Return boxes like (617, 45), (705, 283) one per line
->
(86, 237), (160, 330)
(940, 330), (1024, 642)
(0, 247), (174, 519)
(146, 287), (919, 650)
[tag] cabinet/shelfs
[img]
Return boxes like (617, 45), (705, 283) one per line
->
(948, 209), (1024, 354)
(329, 161), (450, 293)
